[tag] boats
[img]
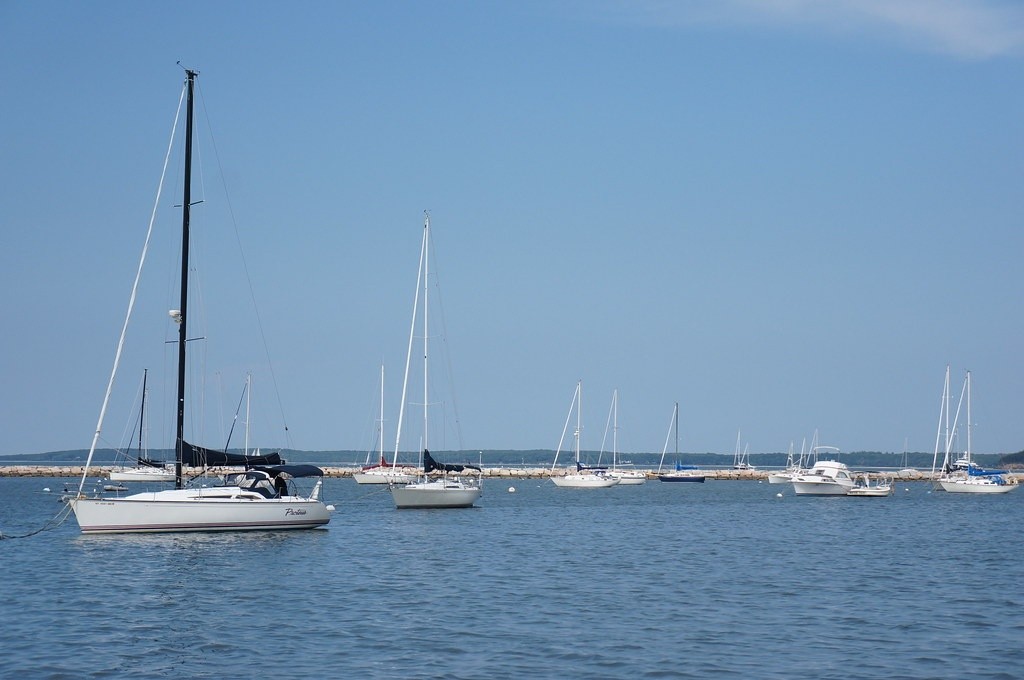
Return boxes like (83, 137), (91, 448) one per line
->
(103, 484), (130, 491)
(110, 369), (176, 482)
(930, 365), (1021, 494)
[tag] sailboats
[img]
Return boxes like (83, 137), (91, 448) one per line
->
(56, 60), (335, 535)
(646, 400), (705, 483)
(597, 387), (646, 485)
(548, 378), (622, 488)
(388, 209), (483, 509)
(353, 365), (417, 484)
(767, 426), (891, 497)
(733, 427), (756, 470)
